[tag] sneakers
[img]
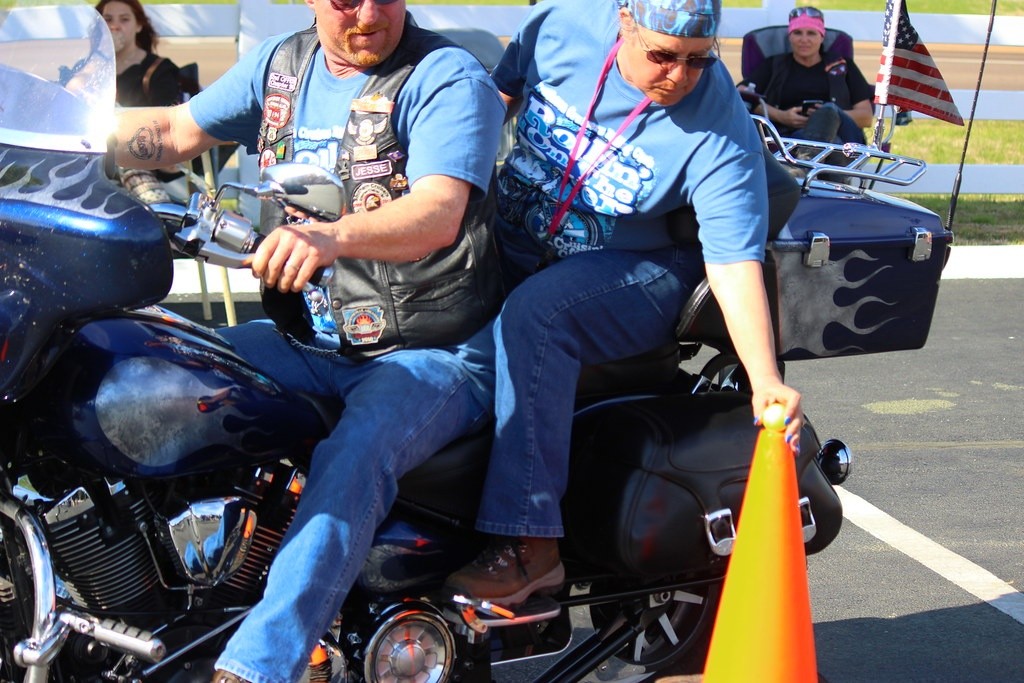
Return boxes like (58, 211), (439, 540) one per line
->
(443, 535), (564, 607)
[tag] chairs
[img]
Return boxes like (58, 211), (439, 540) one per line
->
(742, 25), (854, 83)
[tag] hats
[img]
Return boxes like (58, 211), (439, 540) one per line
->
(614, 0), (723, 37)
(787, 7), (825, 37)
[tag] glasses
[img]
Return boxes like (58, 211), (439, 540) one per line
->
(789, 7), (825, 23)
(330, 0), (398, 12)
(632, 18), (721, 69)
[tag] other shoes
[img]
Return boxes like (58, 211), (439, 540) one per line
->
(778, 161), (817, 179)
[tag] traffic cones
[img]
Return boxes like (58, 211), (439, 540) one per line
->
(654, 403), (823, 683)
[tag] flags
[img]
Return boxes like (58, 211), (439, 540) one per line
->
(874, 0), (965, 126)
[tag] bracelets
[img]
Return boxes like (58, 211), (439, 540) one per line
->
(95, 50), (108, 61)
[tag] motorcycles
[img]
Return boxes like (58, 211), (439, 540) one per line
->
(1, 0), (955, 683)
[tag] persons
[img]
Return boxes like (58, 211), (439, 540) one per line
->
(445, 0), (802, 606)
(734, 5), (875, 185)
(104, 0), (508, 683)
(58, 0), (184, 107)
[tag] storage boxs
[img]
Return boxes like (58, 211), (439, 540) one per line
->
(772, 178), (954, 361)
(561, 392), (844, 586)
